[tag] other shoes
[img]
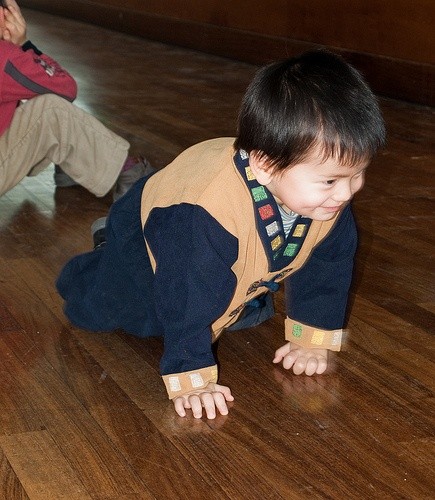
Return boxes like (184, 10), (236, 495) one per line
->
(91, 217), (107, 249)
(113, 153), (161, 202)
(54, 164), (77, 186)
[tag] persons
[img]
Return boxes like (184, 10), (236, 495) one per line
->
(57, 47), (387, 419)
(0, 0), (154, 202)
